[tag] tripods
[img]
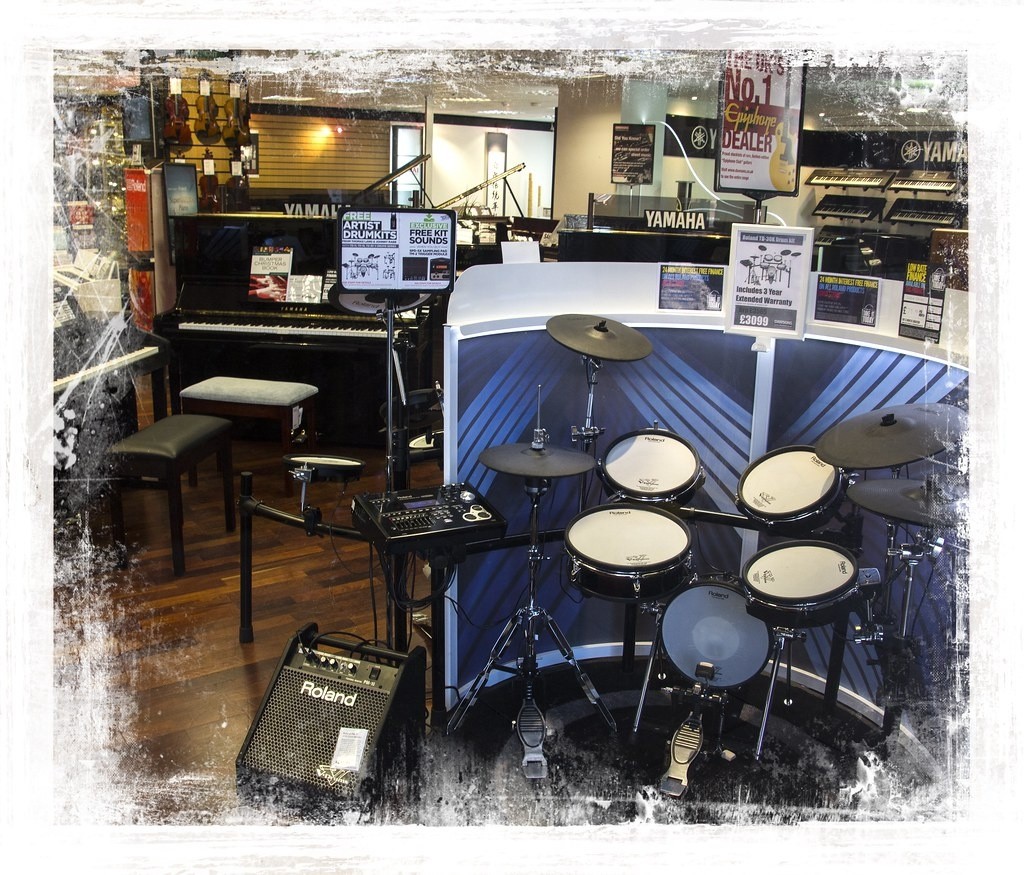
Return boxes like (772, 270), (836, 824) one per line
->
(432, 479), (623, 759)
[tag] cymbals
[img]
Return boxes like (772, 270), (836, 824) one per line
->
(544, 313), (655, 362)
(479, 440), (600, 478)
(845, 477), (968, 528)
(816, 400), (968, 468)
(327, 280), (433, 316)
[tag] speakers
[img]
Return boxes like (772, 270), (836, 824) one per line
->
(234, 621), (427, 826)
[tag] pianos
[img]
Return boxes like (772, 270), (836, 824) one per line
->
(800, 165), (967, 248)
(154, 210), (431, 447)
(349, 151), (560, 243)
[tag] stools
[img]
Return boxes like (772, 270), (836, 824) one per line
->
(108, 414), (236, 576)
(179, 376), (319, 498)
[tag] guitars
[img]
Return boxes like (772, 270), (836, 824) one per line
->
(222, 74), (252, 145)
(193, 75), (221, 137)
(198, 147), (221, 210)
(162, 74), (191, 145)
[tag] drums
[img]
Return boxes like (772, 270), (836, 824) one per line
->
(564, 500), (692, 599)
(407, 427), (444, 449)
(598, 426), (710, 506)
(283, 452), (369, 485)
(740, 535), (860, 624)
(657, 564), (774, 688)
(738, 444), (843, 535)
